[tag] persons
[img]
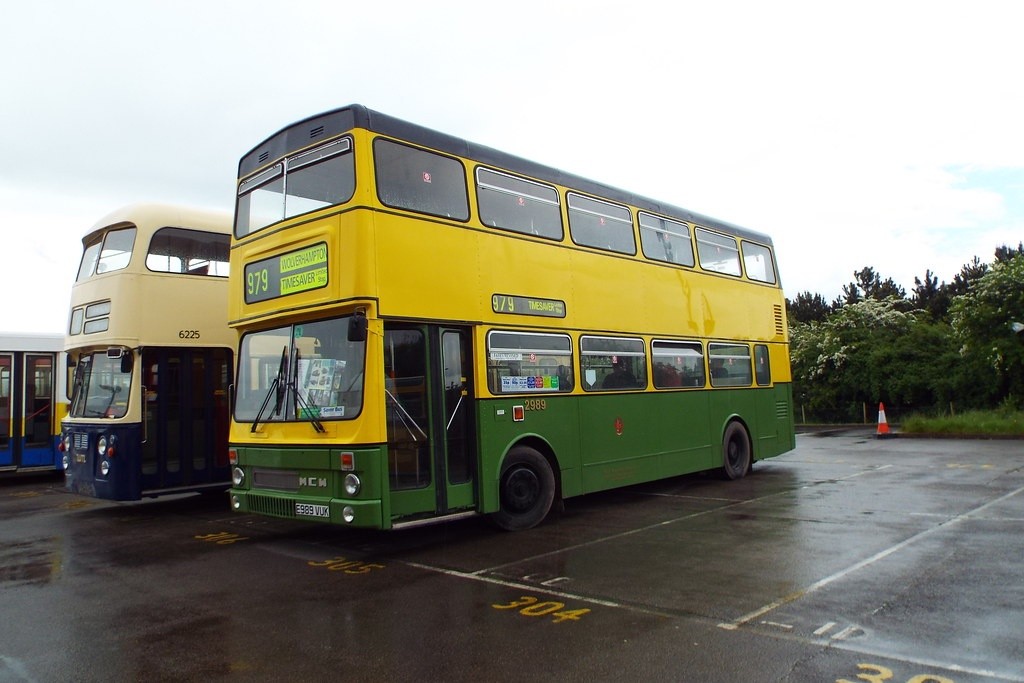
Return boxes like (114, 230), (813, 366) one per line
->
(601, 360), (637, 389)
(653, 358), (703, 386)
(557, 365), (572, 390)
(711, 354), (728, 385)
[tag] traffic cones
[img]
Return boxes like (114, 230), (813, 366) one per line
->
(876, 402), (891, 434)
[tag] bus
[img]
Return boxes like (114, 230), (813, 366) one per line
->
(227, 103), (795, 532)
(0, 334), (66, 474)
(59, 207), (228, 501)
